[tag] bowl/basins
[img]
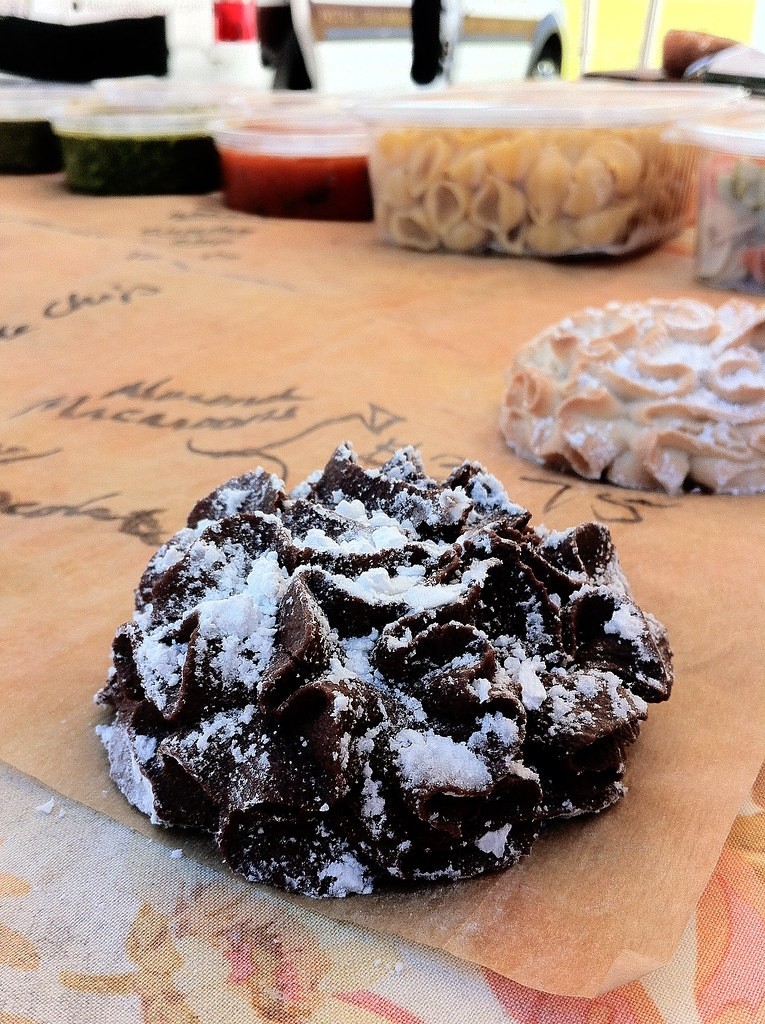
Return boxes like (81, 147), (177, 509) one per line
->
(0, 75), (765, 298)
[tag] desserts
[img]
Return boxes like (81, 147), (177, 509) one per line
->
(498, 300), (764, 499)
(91, 444), (672, 897)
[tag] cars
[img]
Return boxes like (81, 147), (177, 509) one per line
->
(0, 1), (564, 90)
